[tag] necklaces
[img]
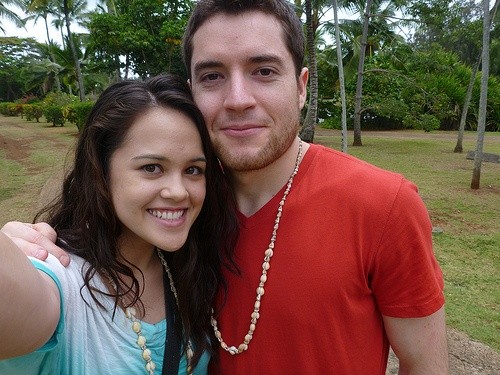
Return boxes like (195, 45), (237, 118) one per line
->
(207, 137), (302, 357)
(98, 246), (194, 375)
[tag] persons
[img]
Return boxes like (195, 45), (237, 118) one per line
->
(0, 0), (449, 375)
(0, 72), (242, 375)
